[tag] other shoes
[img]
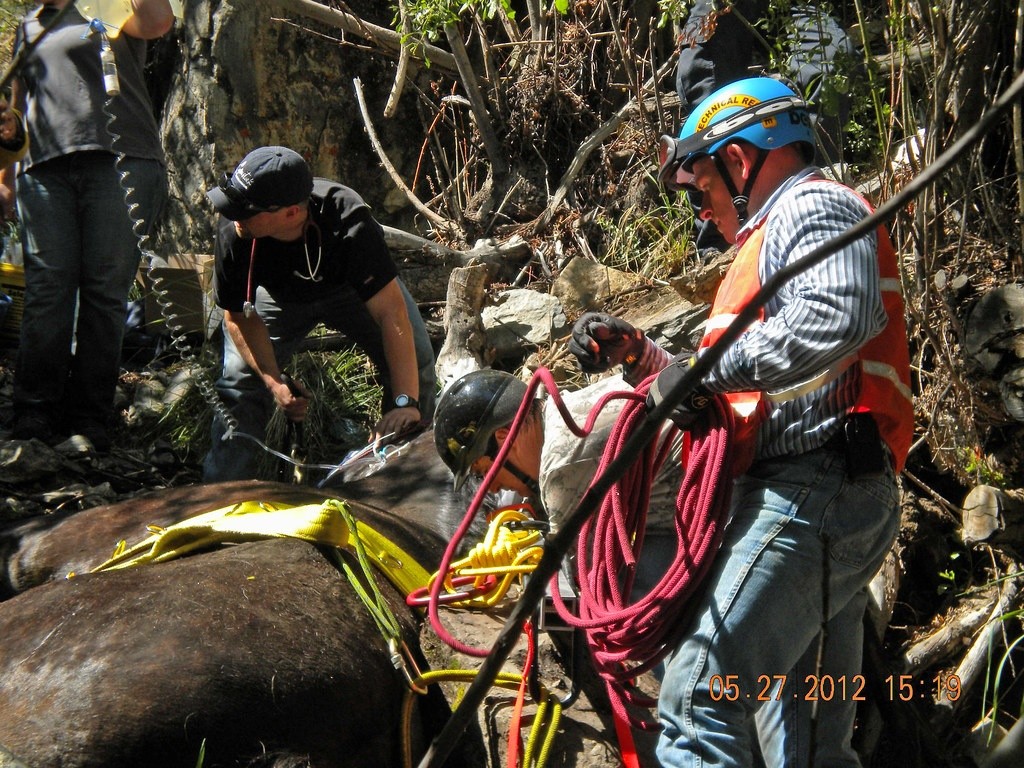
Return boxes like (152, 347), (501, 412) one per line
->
(3, 437), (50, 484)
(52, 434), (132, 492)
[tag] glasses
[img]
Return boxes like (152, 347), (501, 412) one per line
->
(218, 173), (254, 213)
(656, 135), (677, 179)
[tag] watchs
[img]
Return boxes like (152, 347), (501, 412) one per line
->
(393, 395), (419, 408)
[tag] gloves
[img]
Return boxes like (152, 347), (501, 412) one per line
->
(569, 311), (647, 372)
(645, 362), (717, 429)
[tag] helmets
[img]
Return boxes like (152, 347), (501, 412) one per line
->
(659, 77), (821, 175)
(432, 369), (529, 493)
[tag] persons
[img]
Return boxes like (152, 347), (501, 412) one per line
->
(203, 145), (434, 480)
(435, 370), (713, 673)
(0, 0), (176, 459)
(568, 78), (912, 768)
(674, 0), (768, 254)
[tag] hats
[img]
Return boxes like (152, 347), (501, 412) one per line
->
(208, 145), (315, 220)
